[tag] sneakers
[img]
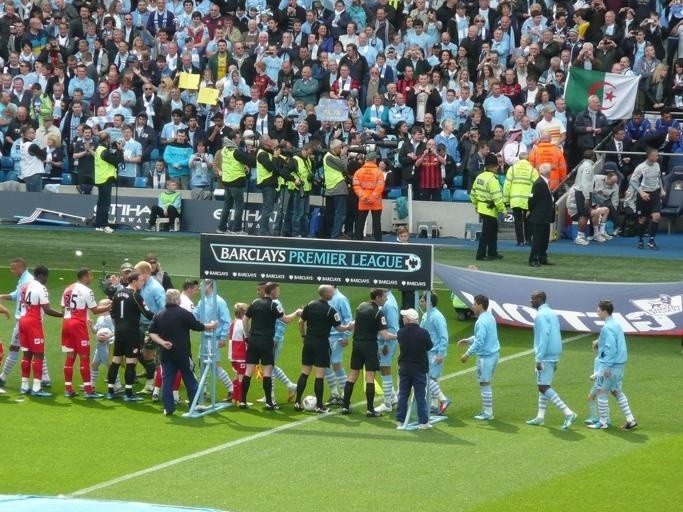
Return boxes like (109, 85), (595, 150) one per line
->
(134, 377), (138, 383)
(341, 404), (353, 414)
(114, 384), (126, 394)
(562, 411), (579, 431)
(584, 417), (598, 424)
(0, 379), (6, 386)
(417, 422), (433, 430)
(323, 395), (340, 407)
(621, 419), (638, 431)
(136, 372), (147, 377)
(164, 408), (168, 416)
(286, 383), (298, 403)
(65, 391), (80, 397)
(41, 380), (52, 387)
(232, 398), (240, 408)
(169, 228), (174, 232)
(196, 404), (204, 411)
(474, 413), (494, 421)
(391, 396), (399, 410)
(145, 226), (156, 232)
(104, 378), (108, 383)
(477, 408), (485, 415)
(103, 226), (115, 233)
(366, 410), (384, 417)
(124, 394), (144, 402)
(223, 390), (234, 401)
(31, 388), (53, 397)
(216, 228), (231, 234)
(374, 403), (393, 412)
(106, 391), (119, 399)
(647, 241), (659, 251)
(429, 407), (438, 413)
(437, 399), (452, 415)
(294, 402), (303, 411)
(20, 387), (33, 394)
(397, 422), (418, 431)
(526, 417), (545, 426)
(0, 388), (5, 394)
(337, 397), (344, 404)
(84, 392), (104, 398)
(92, 384), (95, 389)
(575, 237), (590, 245)
(96, 226), (104, 231)
(202, 392), (207, 400)
(152, 394), (160, 402)
(256, 395), (276, 403)
(136, 387), (155, 396)
(174, 399), (182, 404)
(600, 231), (613, 240)
(593, 234), (605, 243)
(240, 403), (249, 409)
(612, 228), (619, 235)
(314, 406), (332, 414)
(586, 418), (610, 429)
(265, 403), (281, 410)
(231, 230), (249, 236)
(637, 242), (645, 249)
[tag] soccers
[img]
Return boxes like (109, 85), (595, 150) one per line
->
(96, 327), (112, 342)
(303, 395), (316, 411)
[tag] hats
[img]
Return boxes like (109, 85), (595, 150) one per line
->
(432, 42), (443, 48)
(54, 11), (63, 18)
(456, 3), (468, 8)
(531, 10), (543, 17)
(242, 129), (255, 138)
(543, 104), (557, 113)
(400, 307), (420, 322)
(143, 253), (158, 263)
(483, 153), (498, 166)
(126, 54), (138, 62)
(120, 262), (134, 272)
(365, 152), (380, 160)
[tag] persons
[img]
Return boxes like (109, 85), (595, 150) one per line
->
(271, 141), (292, 237)
(395, 226), (416, 308)
(502, 152), (539, 247)
(388, 0), (478, 198)
(419, 291), (451, 415)
(0, 257), (52, 387)
(192, 278), (234, 402)
(19, 265), (64, 397)
(478, 0), (568, 151)
(252, 283), (297, 403)
(257, 134), (281, 235)
(144, 179), (182, 233)
(526, 290), (579, 431)
(149, 288), (217, 415)
(586, 300), (638, 430)
(111, 261), (166, 395)
(396, 308), (433, 430)
(280, 143), (314, 237)
(95, 1), (322, 148)
(61, 270), (112, 399)
(352, 289), (398, 413)
(525, 163), (556, 267)
(162, 129), (194, 190)
(88, 298), (122, 393)
(315, 0), (388, 241)
(188, 136), (214, 191)
(152, 279), (198, 405)
(144, 253), (173, 292)
(229, 303), (249, 408)
(573, 149), (595, 247)
(0, 303), (11, 367)
(592, 173), (620, 236)
(120, 125), (142, 187)
(469, 153), (507, 261)
(418, 316), (446, 322)
(0, 1), (94, 196)
(110, 273), (156, 402)
(216, 130), (256, 235)
(342, 288), (392, 417)
(147, 158), (166, 189)
(239, 282), (303, 410)
(568, 0), (683, 148)
(451, 264), (480, 320)
(630, 149), (666, 250)
(457, 295), (500, 420)
(94, 132), (122, 234)
(584, 341), (612, 426)
(618, 183), (638, 236)
(566, 184), (612, 242)
(101, 263), (134, 299)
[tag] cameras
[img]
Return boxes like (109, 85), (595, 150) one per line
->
(279, 146), (295, 157)
(306, 171), (322, 186)
(346, 144), (365, 154)
(366, 139), (399, 149)
(112, 139), (126, 147)
(296, 180), (308, 200)
(239, 139), (261, 148)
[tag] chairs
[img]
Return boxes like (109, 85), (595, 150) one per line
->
(1, 156), (16, 170)
(6, 171), (23, 183)
(453, 189), (475, 203)
(134, 176), (149, 188)
(386, 189), (401, 200)
(453, 175), (470, 189)
(431, 190), (450, 202)
(62, 174), (75, 185)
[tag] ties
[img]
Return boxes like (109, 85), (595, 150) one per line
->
(617, 143), (624, 167)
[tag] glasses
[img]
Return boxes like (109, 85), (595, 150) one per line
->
(213, 119), (221, 122)
(238, 46), (244, 50)
(96, 37), (100, 40)
(20, 65), (24, 67)
(433, 47), (440, 49)
(125, 18), (132, 20)
(144, 87), (153, 91)
(129, 61), (135, 63)
(54, 17), (62, 19)
(161, 80), (166, 84)
(475, 19), (484, 23)
(470, 126), (479, 130)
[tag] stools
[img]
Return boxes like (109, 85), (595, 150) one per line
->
(155, 217), (181, 232)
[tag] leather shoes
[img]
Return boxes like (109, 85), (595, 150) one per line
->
(524, 241), (531, 246)
(488, 253), (503, 259)
(516, 242), (524, 247)
(540, 258), (556, 266)
(529, 260), (541, 267)
(477, 255), (493, 261)
